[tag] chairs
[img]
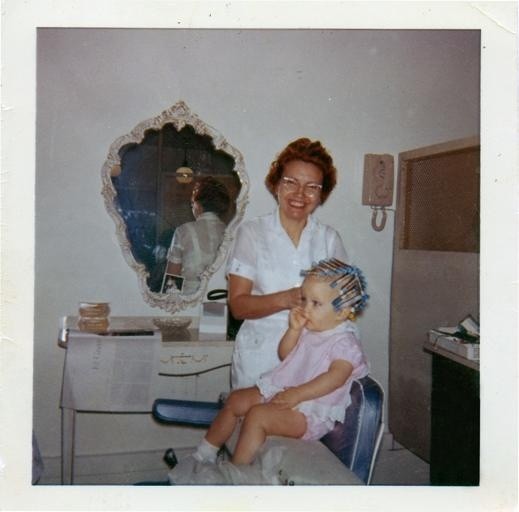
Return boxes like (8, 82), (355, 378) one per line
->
(151, 369), (387, 488)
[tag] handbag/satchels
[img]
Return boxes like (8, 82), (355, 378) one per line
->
(208, 290), (244, 340)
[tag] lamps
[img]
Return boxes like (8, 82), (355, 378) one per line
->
(110, 164), (122, 178)
(174, 142), (194, 186)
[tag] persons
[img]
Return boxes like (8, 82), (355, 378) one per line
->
(162, 276), (180, 294)
(165, 175), (230, 295)
(192, 256), (370, 466)
(225, 136), (347, 393)
(148, 228), (174, 293)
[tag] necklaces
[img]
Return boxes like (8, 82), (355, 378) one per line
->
(284, 220), (307, 247)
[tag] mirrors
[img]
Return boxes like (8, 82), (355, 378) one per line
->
(99, 99), (250, 316)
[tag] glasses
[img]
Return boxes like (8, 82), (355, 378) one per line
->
(280, 176), (323, 198)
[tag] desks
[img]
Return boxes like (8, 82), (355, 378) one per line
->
(56, 315), (244, 485)
(422, 326), (479, 485)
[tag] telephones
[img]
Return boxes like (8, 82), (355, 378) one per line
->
(362, 154), (394, 206)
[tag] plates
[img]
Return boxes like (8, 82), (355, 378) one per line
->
(153, 316), (192, 330)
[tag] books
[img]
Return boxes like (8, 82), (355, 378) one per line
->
(428, 330), (479, 362)
